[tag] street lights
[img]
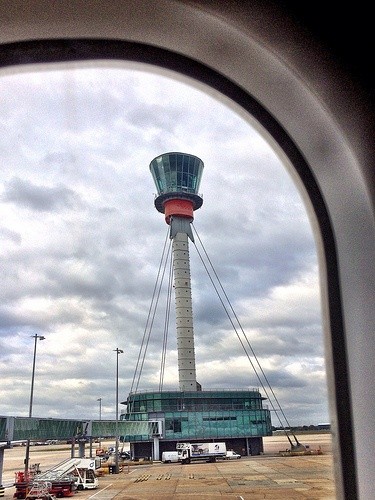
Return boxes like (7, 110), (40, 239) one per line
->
(97, 398), (102, 450)
(24, 332), (46, 473)
(113, 347), (125, 475)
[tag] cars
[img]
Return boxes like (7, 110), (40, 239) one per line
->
(0, 438), (100, 447)
(93, 446), (152, 465)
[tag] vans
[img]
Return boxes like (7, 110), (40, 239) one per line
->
(223, 450), (242, 460)
(161, 451), (182, 464)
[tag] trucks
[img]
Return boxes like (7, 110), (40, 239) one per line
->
(176, 441), (227, 465)
